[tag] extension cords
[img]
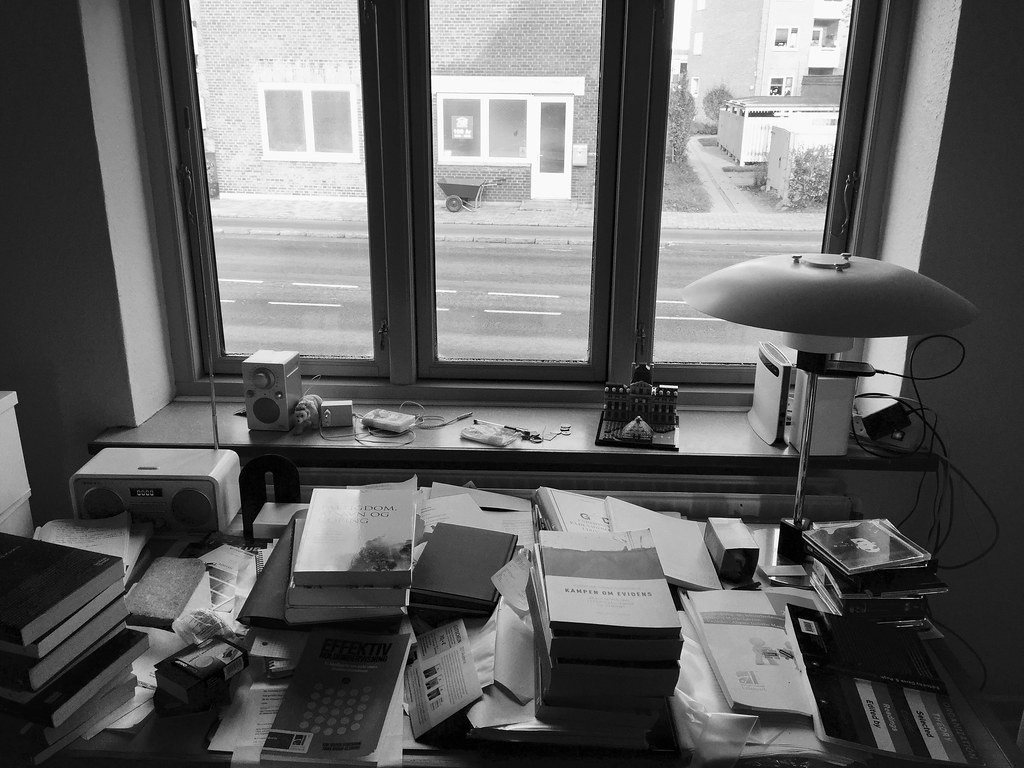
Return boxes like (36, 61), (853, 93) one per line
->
(850, 413), (923, 453)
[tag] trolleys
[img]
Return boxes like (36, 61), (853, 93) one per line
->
(436, 174), (509, 214)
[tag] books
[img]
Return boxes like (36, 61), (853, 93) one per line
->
(409, 522), (524, 615)
(235, 489), (417, 633)
(260, 628), (413, 768)
(33, 516), (153, 587)
(784, 603), (985, 768)
(679, 590), (833, 717)
(197, 543), (256, 614)
(0, 532), (150, 765)
(532, 487), (723, 591)
(525, 529), (684, 729)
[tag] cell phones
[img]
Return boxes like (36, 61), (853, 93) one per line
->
(825, 360), (875, 377)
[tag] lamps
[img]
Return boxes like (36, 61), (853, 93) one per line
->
(680, 253), (979, 590)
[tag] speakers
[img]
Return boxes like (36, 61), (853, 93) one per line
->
(242, 349), (301, 432)
(69, 448), (241, 541)
